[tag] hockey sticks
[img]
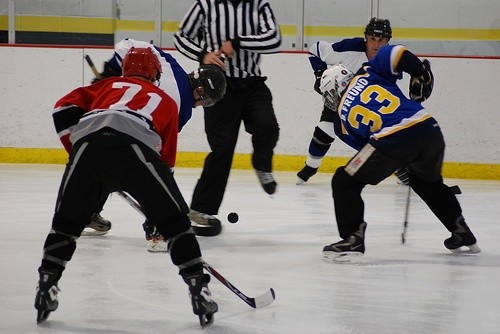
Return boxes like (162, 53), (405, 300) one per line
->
(84, 53), (222, 237)
(401, 58), (431, 245)
(114, 186), (276, 308)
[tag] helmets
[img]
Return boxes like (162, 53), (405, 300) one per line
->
(193, 65), (227, 105)
(121, 46), (161, 82)
(364, 16), (393, 39)
(319, 63), (354, 111)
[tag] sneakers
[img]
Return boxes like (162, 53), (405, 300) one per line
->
(184, 273), (218, 326)
(188, 210), (220, 228)
(255, 168), (277, 195)
(35, 267), (62, 322)
(80, 213), (111, 235)
(142, 220), (168, 252)
(323, 222), (368, 262)
(297, 162), (319, 184)
(443, 217), (481, 252)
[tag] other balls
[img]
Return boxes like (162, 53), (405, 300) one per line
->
(227, 212), (239, 224)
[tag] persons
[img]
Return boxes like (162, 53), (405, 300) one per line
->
(35, 46), (218, 329)
(80, 38), (227, 252)
(319, 44), (481, 264)
(174, 0), (282, 226)
(296, 19), (393, 185)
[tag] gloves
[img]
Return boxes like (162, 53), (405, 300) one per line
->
(314, 70), (327, 95)
(409, 60), (435, 102)
(92, 62), (118, 83)
